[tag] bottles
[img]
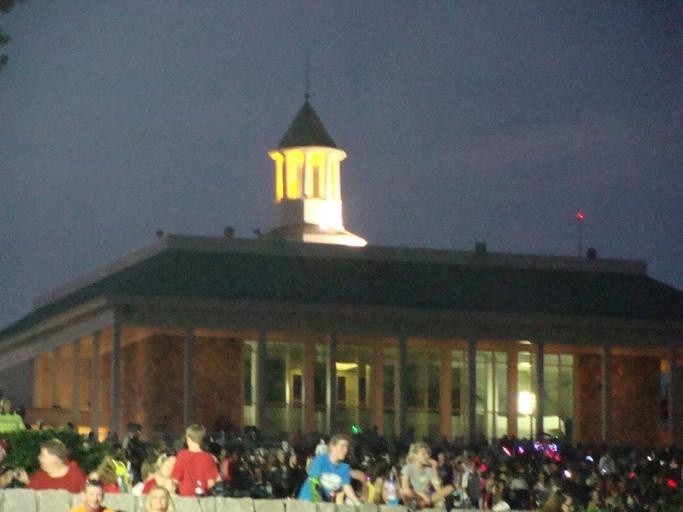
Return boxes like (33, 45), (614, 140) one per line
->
(360, 477), (375, 504)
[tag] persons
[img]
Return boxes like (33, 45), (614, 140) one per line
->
(0, 396), (683, 511)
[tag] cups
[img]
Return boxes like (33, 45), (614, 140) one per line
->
(382, 478), (400, 506)
